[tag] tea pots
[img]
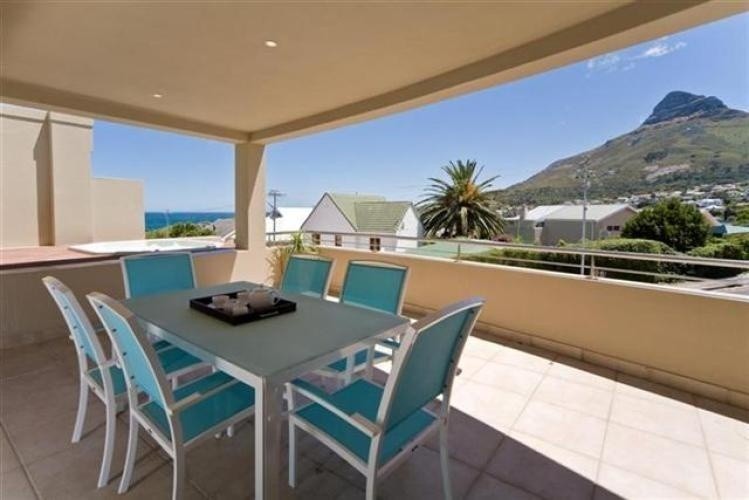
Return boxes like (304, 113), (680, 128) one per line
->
(239, 284), (281, 308)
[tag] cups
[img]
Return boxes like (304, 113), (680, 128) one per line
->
(212, 292), (251, 315)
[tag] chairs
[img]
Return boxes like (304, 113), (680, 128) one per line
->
(42, 275), (221, 488)
(85, 291), (254, 500)
(279, 253), (336, 299)
(119, 251), (197, 298)
(313, 258), (411, 387)
(282, 295), (487, 500)
